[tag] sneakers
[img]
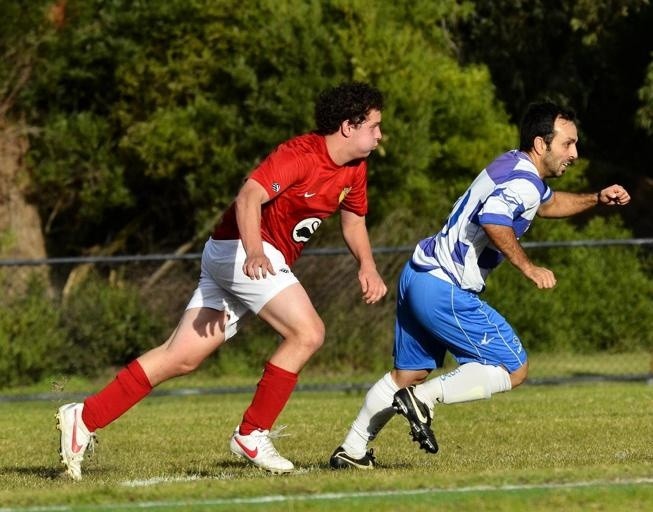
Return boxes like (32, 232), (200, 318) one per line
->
(330, 446), (386, 469)
(55, 402), (96, 481)
(392, 386), (438, 453)
(228, 425), (294, 472)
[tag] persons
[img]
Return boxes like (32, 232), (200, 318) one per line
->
(328, 98), (631, 470)
(52, 81), (390, 481)
